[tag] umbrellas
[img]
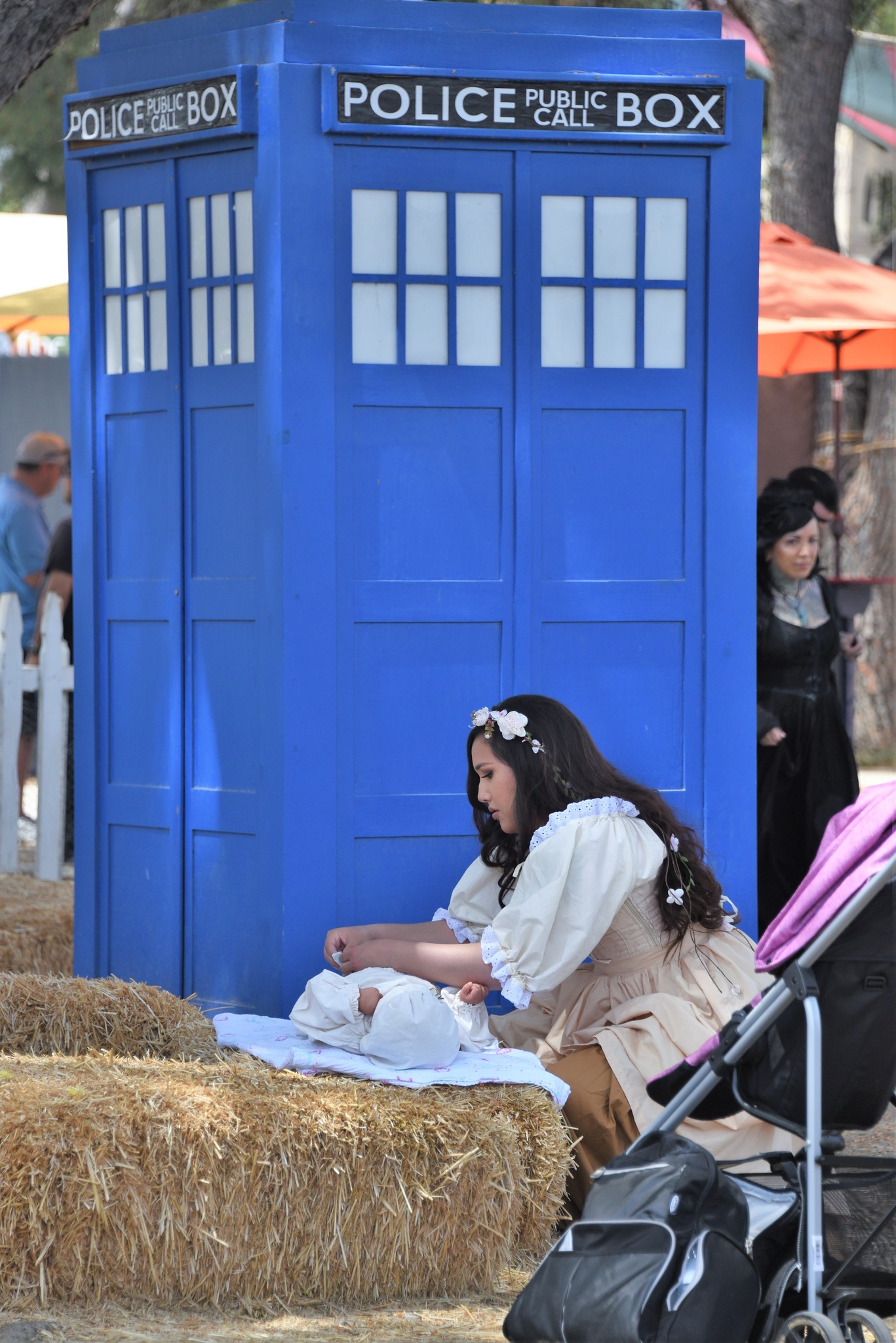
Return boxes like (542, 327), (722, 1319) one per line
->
(758, 224), (896, 605)
(0, 210), (69, 360)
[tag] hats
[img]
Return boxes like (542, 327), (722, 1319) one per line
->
(17, 435), (67, 469)
(359, 985), (460, 1070)
(761, 487), (814, 536)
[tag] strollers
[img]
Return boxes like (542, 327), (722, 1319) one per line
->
(503, 780), (896, 1342)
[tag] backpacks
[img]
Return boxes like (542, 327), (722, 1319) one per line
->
(503, 1131), (760, 1343)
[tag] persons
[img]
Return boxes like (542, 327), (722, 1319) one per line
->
(757, 466), (861, 944)
(323, 693), (851, 1236)
(1, 431), (75, 884)
(289, 966), (489, 1072)
